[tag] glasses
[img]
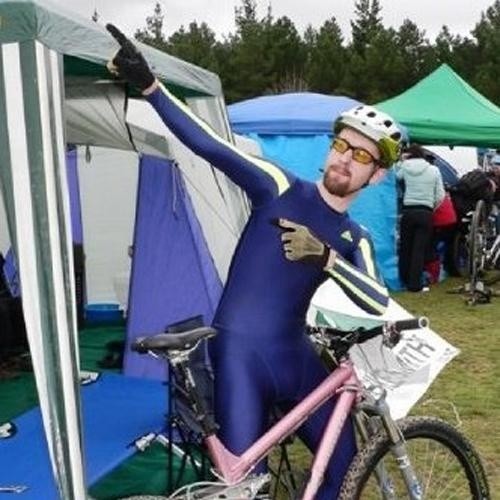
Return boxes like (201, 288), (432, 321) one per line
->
(333, 136), (386, 168)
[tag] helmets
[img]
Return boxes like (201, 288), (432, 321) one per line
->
(332, 103), (405, 162)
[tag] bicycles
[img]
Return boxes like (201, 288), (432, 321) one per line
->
(445, 195), (499, 308)
(121, 316), (489, 499)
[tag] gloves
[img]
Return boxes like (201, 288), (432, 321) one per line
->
(271, 217), (330, 270)
(105, 24), (155, 92)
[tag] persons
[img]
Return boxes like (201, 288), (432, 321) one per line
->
(103, 21), (405, 500)
(393, 143), (500, 293)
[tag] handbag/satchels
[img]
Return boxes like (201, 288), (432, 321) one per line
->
(432, 192), (458, 228)
